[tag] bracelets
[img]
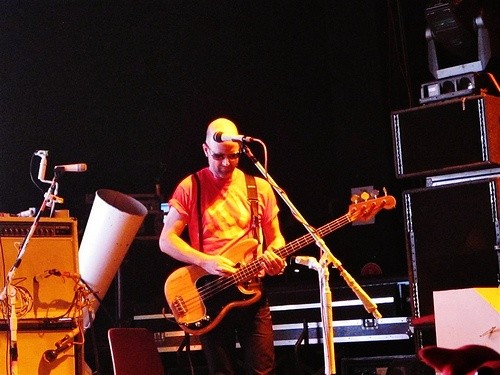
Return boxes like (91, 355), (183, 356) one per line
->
(278, 261), (288, 275)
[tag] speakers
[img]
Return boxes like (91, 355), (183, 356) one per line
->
(390, 93), (500, 178)
(0, 326), (84, 375)
(96, 237), (185, 331)
(400, 175), (500, 324)
(0, 216), (79, 328)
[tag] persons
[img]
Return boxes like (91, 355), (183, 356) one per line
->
(159, 118), (287, 375)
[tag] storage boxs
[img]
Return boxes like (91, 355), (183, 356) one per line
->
(390, 93), (500, 181)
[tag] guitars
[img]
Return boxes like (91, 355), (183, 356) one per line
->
(163, 188), (397, 336)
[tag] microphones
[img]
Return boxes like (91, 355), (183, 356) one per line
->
(54, 163), (87, 171)
(213, 132), (252, 142)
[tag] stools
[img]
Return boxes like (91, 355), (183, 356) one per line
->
(418, 345), (500, 375)
(411, 314), (435, 349)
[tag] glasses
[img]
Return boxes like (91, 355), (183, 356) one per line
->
(205, 141), (241, 161)
